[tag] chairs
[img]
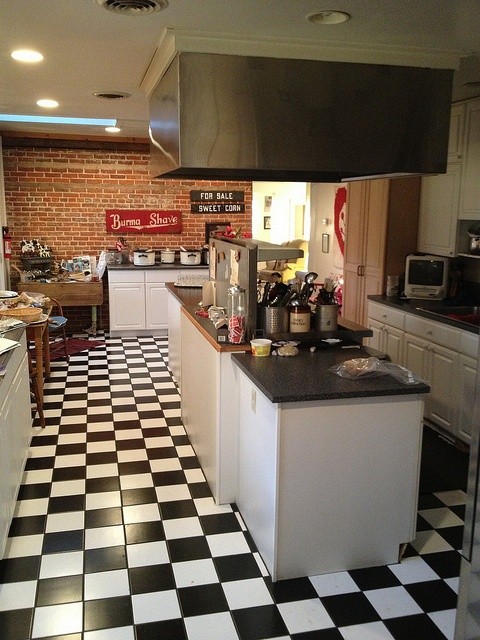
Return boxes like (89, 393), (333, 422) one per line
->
(27, 297), (69, 364)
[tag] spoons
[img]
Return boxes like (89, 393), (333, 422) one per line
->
(331, 286), (339, 303)
(291, 299), (302, 306)
(270, 273), (282, 285)
(303, 272), (318, 284)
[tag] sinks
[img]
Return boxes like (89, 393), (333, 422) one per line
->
(422, 305), (480, 328)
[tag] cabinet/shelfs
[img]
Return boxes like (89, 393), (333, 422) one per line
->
(456, 327), (480, 447)
(416, 100), (467, 259)
(108, 267), (211, 330)
(342, 175), (423, 347)
(405, 315), (459, 438)
(457, 97), (480, 219)
(365, 300), (404, 365)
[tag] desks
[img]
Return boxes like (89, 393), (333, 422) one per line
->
(18, 277), (103, 338)
(0, 305), (54, 428)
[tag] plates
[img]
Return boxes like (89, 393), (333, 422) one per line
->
(0, 290), (18, 297)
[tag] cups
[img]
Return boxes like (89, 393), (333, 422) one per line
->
(250, 337), (273, 358)
(227, 283), (246, 344)
(290, 304), (310, 332)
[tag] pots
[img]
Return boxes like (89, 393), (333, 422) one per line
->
(133, 249), (156, 266)
(179, 248), (202, 266)
(160, 249), (176, 263)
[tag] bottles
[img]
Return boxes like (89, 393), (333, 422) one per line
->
(387, 275), (399, 297)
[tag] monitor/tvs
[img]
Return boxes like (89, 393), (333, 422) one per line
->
(404, 254), (448, 300)
